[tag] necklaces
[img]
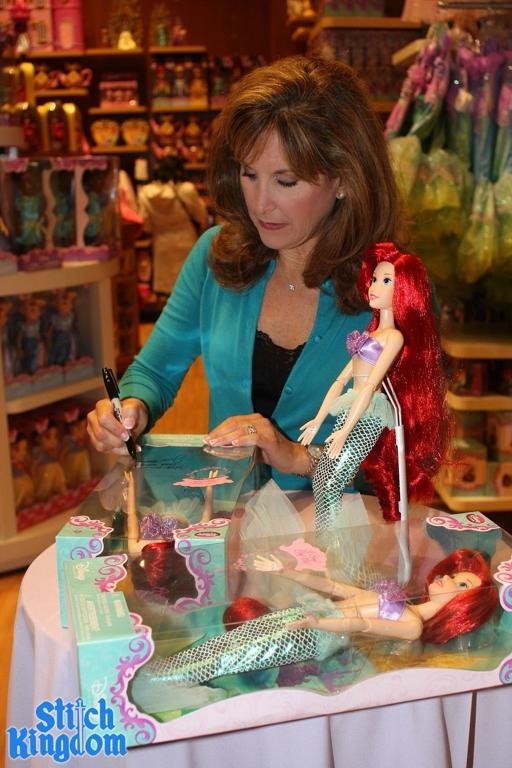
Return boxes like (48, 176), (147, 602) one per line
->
(277, 261), (313, 294)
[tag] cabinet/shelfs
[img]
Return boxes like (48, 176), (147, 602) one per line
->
(431, 332), (512, 514)
(1, 46), (211, 576)
(307, 18), (422, 111)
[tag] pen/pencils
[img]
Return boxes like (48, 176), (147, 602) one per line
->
(102, 367), (135, 458)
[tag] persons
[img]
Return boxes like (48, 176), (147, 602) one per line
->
(1, 0), (274, 516)
(83, 53), (442, 502)
(297, 237), (457, 535)
(132, 542), (499, 691)
(0, 1), (272, 515)
(119, 466), (226, 615)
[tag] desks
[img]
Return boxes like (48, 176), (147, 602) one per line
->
(2, 491), (509, 766)
